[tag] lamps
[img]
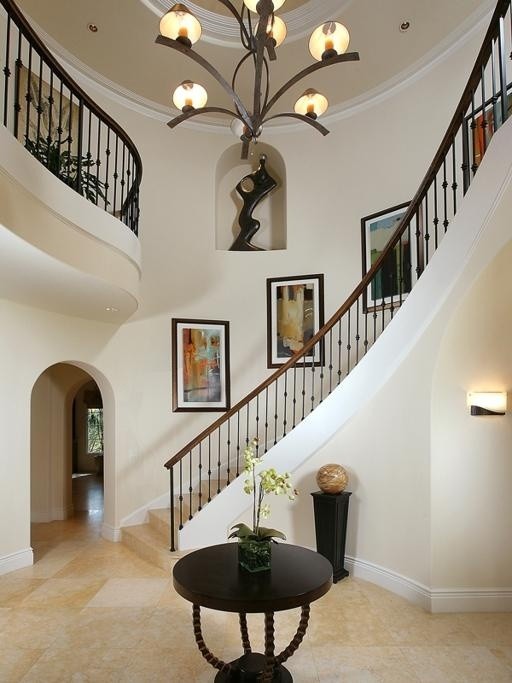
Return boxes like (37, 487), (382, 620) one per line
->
(155, 0), (360, 160)
(468, 390), (507, 416)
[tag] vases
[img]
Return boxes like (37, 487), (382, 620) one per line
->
(239, 537), (273, 573)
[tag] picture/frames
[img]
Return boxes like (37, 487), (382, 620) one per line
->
(266, 273), (325, 369)
(172, 318), (230, 412)
(361, 200), (423, 314)
(472, 93), (512, 170)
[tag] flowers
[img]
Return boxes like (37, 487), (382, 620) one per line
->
(228, 438), (295, 544)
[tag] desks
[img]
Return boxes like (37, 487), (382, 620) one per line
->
(173, 542), (333, 683)
